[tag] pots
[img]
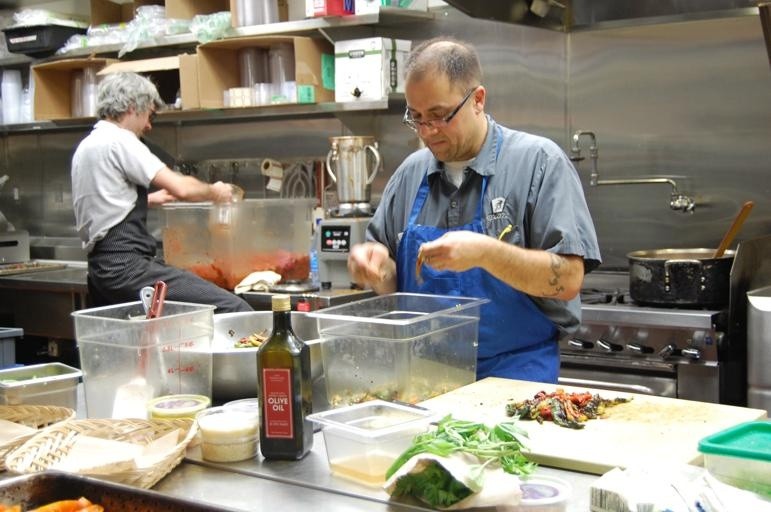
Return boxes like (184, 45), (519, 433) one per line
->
(625, 244), (739, 306)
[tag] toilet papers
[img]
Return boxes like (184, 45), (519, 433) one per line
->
(259, 159), (283, 193)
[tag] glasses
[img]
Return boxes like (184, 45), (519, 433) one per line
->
(401, 86), (478, 131)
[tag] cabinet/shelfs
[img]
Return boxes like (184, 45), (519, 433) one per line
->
(0, 12), (415, 137)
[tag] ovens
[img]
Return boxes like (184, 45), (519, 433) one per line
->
(559, 322), (720, 403)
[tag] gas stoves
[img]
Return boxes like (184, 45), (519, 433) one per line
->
(577, 287), (731, 312)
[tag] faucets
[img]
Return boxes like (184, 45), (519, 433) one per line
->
(572, 128), (599, 184)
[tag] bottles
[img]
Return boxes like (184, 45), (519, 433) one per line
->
(255, 292), (316, 463)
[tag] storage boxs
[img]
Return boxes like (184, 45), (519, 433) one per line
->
(161, 195), (318, 290)
(30, 34), (412, 120)
(89, 0), (237, 29)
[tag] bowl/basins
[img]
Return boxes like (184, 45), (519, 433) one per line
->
(159, 308), (321, 406)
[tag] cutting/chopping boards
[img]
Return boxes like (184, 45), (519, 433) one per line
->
(412, 374), (768, 483)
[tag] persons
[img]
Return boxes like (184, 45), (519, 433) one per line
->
(69, 71), (257, 378)
(348, 35), (606, 386)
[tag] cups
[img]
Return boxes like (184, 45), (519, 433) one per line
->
(269, 45), (296, 103)
(1, 65), (117, 125)
(238, 46), (269, 109)
(207, 184), (247, 239)
(321, 132), (381, 217)
